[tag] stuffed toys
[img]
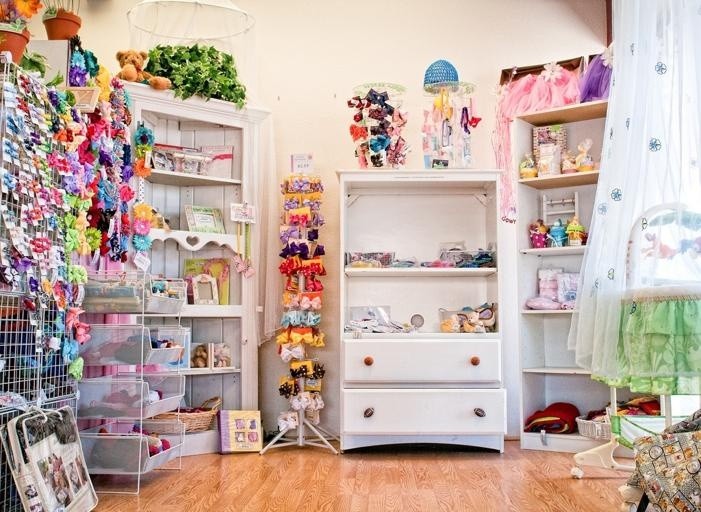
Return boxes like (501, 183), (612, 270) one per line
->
(113, 48), (174, 93)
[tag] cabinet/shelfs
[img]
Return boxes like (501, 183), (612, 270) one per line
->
(73, 273), (190, 494)
(121, 78), (262, 466)
(507, 98), (662, 450)
(335, 169), (508, 458)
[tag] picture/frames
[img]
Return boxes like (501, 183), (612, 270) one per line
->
(192, 274), (222, 306)
(183, 204), (227, 235)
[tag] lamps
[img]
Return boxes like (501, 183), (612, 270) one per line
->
(424, 58), (460, 169)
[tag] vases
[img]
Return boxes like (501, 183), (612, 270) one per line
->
(45, 13), (83, 43)
(0, 20), (31, 67)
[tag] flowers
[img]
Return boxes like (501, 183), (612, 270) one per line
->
(0, 1), (43, 31)
